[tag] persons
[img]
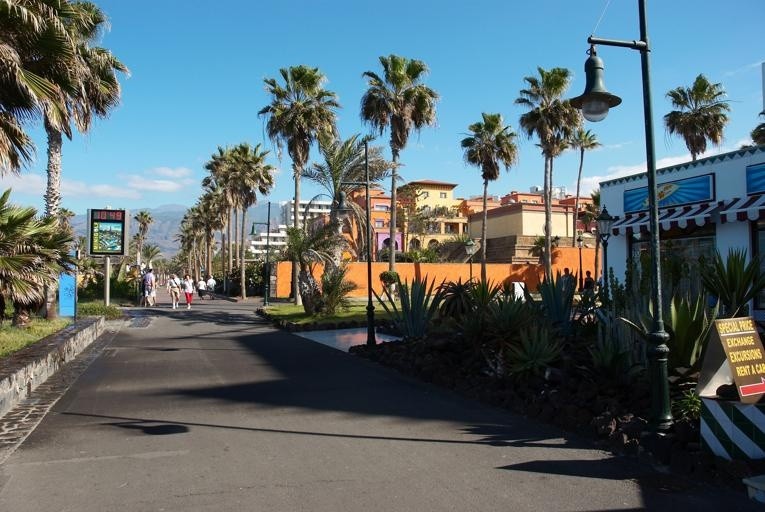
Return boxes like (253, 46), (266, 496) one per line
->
(139, 269), (217, 310)
(599, 269), (602, 287)
(561, 267), (574, 317)
(583, 270), (594, 302)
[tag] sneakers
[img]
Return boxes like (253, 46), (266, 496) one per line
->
(173, 302), (179, 309)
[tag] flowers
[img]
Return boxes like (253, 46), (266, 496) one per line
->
(348, 340), (392, 363)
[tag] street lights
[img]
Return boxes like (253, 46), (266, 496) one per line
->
(336, 138), (376, 348)
(464, 236), (475, 285)
(248, 202), (272, 306)
(570, 3), (676, 430)
